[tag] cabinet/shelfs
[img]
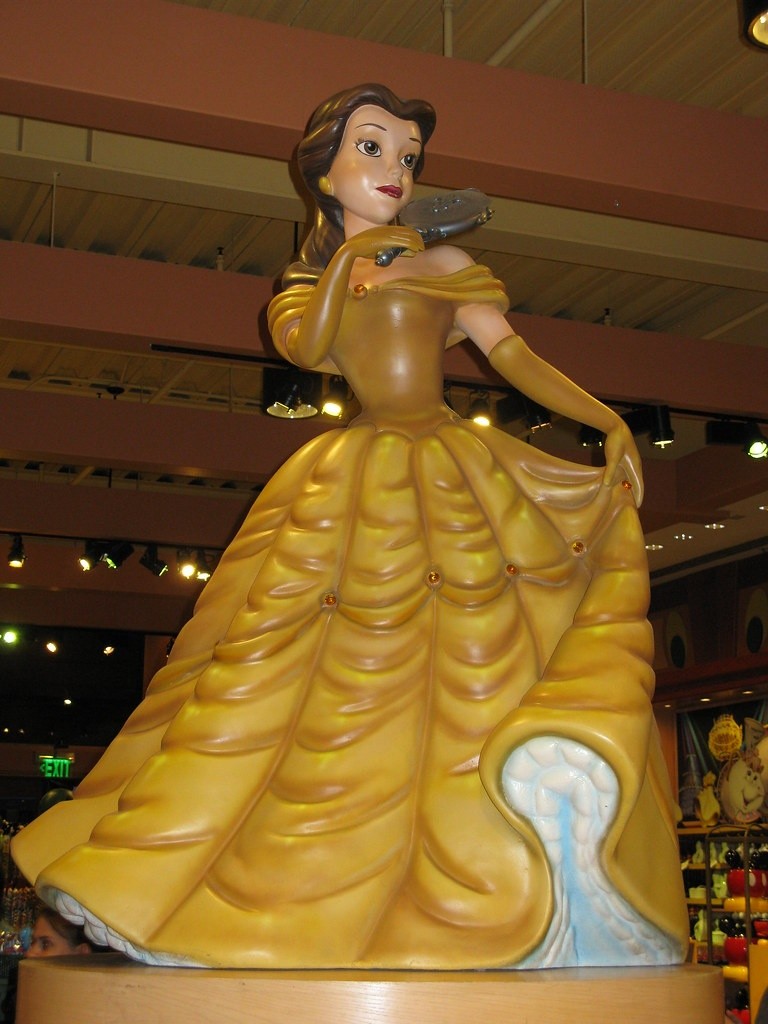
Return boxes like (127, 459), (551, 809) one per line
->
(676, 828), (768, 1024)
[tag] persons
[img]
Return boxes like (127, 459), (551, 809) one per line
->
(9, 84), (690, 970)
(24, 908), (91, 958)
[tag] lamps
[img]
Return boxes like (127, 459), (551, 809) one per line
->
(576, 425), (603, 448)
(7, 536), (26, 568)
(495, 392), (553, 433)
(275, 374), (308, 412)
(261, 370), (318, 420)
(321, 376), (355, 417)
(468, 385), (492, 428)
(196, 551), (212, 582)
(140, 546), (168, 577)
(621, 405), (674, 449)
(79, 543), (106, 570)
(106, 543), (135, 570)
(705, 421), (768, 459)
(175, 548), (196, 581)
(742, 0), (768, 51)
